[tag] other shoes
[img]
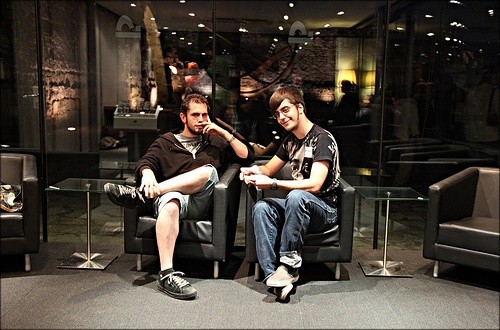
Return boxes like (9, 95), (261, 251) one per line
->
(265, 265), (300, 287)
(273, 283), (294, 301)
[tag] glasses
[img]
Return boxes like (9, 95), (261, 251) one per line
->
(273, 102), (298, 120)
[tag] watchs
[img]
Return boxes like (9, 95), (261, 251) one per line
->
(271, 179), (278, 191)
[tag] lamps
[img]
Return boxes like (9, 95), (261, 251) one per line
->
(237, 18), (249, 33)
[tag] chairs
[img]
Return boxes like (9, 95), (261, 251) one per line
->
(0, 119), (500, 280)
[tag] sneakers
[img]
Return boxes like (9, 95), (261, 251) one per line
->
(156, 268), (198, 299)
(104, 183), (147, 209)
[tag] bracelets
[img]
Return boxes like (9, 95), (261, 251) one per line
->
(232, 130), (236, 133)
(250, 143), (254, 146)
(227, 135), (235, 143)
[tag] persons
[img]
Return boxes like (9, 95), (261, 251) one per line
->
(340, 80), (418, 187)
(103, 94), (255, 300)
(214, 117), (285, 157)
(239, 86), (340, 302)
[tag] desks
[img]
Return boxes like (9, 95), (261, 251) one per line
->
(353, 185), (430, 278)
(97, 158), (139, 232)
(44, 178), (126, 271)
